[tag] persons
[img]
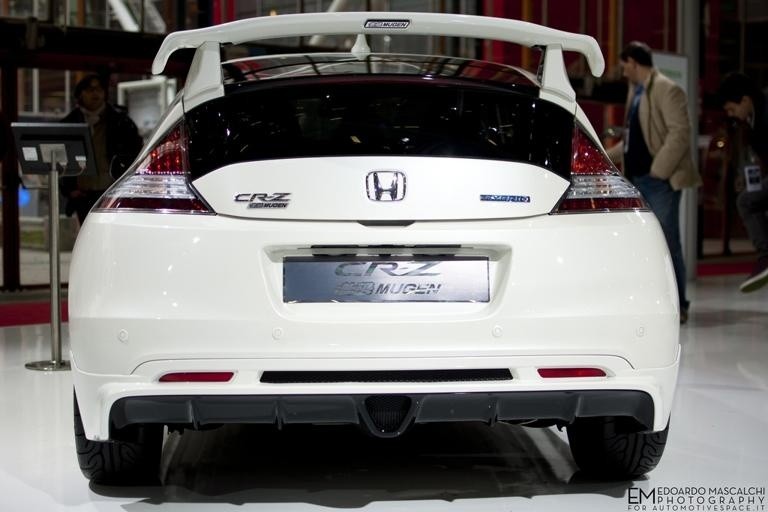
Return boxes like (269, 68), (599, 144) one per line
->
(605, 40), (701, 324)
(57, 72), (142, 227)
(719, 72), (767, 294)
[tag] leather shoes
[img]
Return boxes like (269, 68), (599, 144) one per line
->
(679, 305), (688, 324)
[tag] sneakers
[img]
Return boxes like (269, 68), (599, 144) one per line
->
(740, 256), (768, 292)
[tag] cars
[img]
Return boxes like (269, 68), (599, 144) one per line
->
(63, 9), (686, 487)
